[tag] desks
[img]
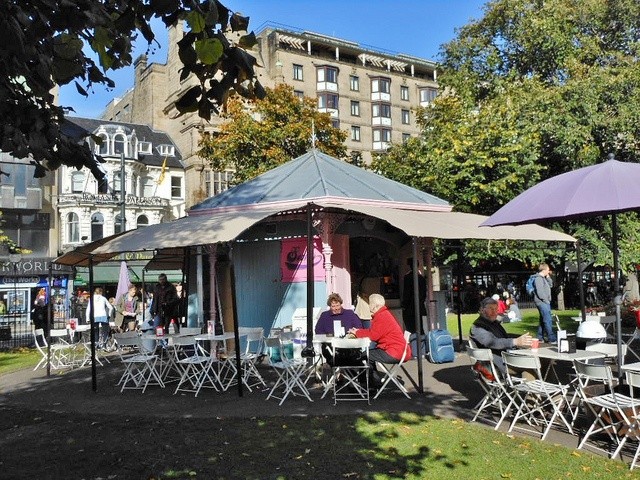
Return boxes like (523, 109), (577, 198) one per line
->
(508, 345), (607, 433)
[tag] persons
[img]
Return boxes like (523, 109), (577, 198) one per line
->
(348, 293), (413, 396)
(401, 257), (428, 335)
(32, 290), (46, 337)
(116, 285), (141, 334)
(165, 297), (180, 334)
(105, 297), (115, 321)
(491, 294), (507, 316)
(621, 262), (639, 329)
(154, 273), (177, 335)
(468, 297), (536, 382)
(86, 288), (115, 351)
(315, 293), (367, 386)
(534, 263), (558, 344)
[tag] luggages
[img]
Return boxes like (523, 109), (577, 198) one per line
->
(427, 328), (455, 364)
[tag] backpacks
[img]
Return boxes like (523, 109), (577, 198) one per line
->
(526, 274), (545, 298)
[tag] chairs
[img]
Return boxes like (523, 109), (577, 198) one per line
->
(501, 351), (573, 441)
(566, 342), (627, 420)
(611, 371), (640, 471)
(331, 337), (371, 407)
(573, 360), (640, 461)
(467, 346), (525, 427)
(556, 315), (576, 340)
(588, 316), (617, 343)
(267, 328), (328, 406)
(112, 327), (266, 399)
(614, 324), (640, 363)
(33, 319), (116, 371)
(290, 333), (363, 386)
(372, 330), (412, 400)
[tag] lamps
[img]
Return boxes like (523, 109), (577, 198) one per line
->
(576, 315), (606, 344)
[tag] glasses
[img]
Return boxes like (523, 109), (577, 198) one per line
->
(485, 308), (499, 312)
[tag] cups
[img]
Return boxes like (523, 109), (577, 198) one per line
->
(530, 338), (539, 352)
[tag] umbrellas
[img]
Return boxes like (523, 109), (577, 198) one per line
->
(478, 153), (640, 393)
